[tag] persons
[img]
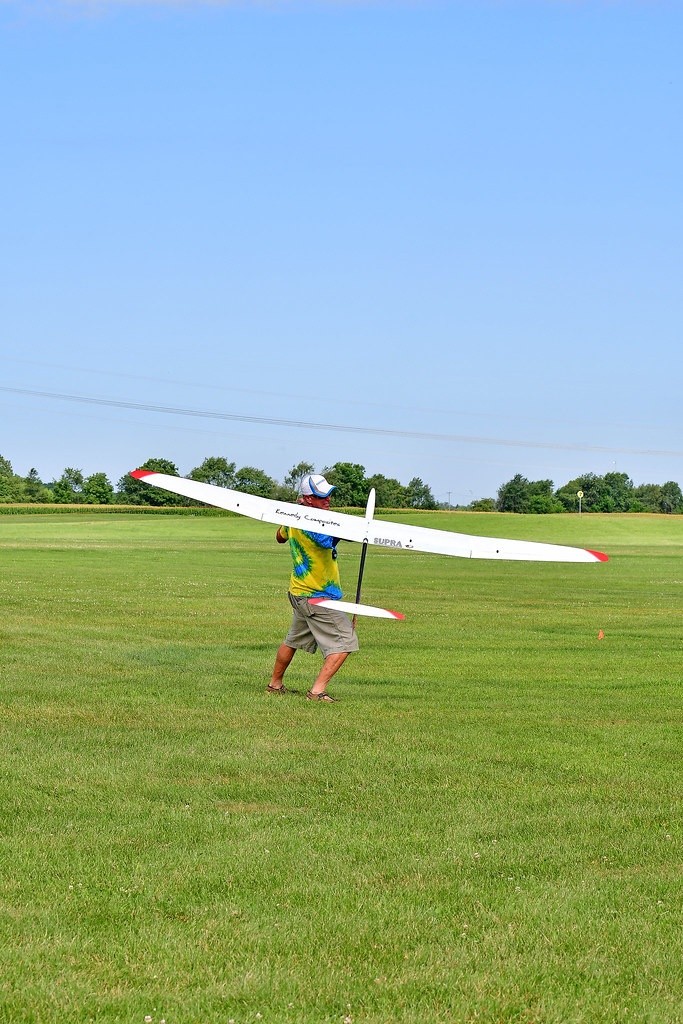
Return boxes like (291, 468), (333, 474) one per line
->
(266, 475), (360, 703)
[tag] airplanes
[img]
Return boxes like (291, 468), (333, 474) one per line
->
(129, 469), (610, 635)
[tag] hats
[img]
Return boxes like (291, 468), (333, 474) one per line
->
(301, 475), (337, 497)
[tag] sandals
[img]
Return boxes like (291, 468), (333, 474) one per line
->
(264, 684), (299, 695)
(307, 689), (340, 703)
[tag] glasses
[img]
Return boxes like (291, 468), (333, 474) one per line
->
(313, 492), (332, 499)
